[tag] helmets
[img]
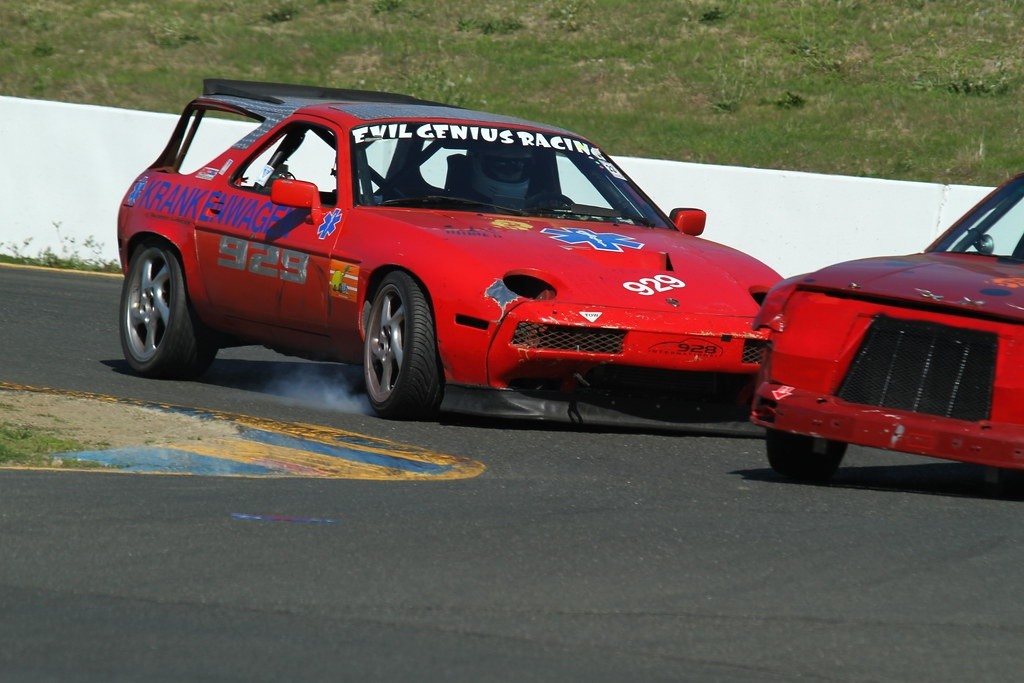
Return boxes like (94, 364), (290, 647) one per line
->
(470, 139), (533, 197)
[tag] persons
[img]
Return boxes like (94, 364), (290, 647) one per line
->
(468, 147), (534, 199)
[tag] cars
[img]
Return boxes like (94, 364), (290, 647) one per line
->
(751, 172), (1024, 479)
(117, 78), (787, 422)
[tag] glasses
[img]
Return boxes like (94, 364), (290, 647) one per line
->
(487, 158), (525, 173)
